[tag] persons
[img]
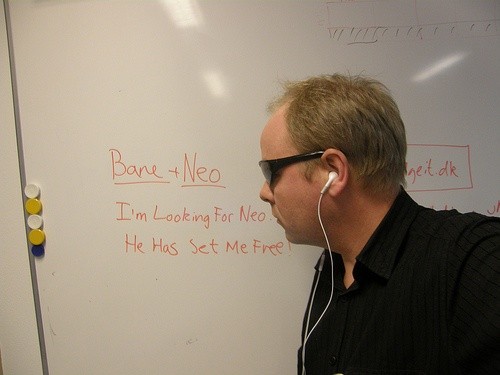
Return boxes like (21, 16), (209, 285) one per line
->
(259, 72), (500, 374)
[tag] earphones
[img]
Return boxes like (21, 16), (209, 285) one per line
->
(320, 171), (339, 196)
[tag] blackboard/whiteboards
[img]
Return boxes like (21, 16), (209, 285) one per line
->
(2, 1), (499, 375)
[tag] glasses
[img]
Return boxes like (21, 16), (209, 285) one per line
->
(259, 151), (326, 186)
